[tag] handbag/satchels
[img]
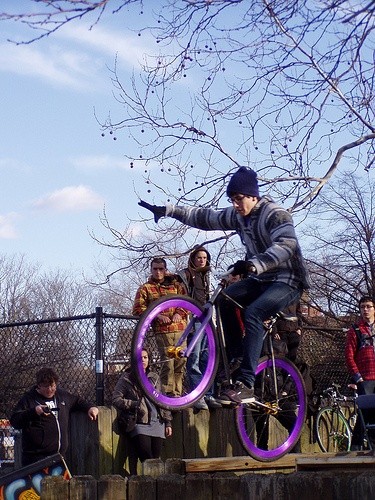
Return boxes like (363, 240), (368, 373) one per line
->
(113, 409), (137, 435)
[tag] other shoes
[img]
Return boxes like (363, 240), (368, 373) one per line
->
(350, 445), (359, 451)
(206, 396), (222, 407)
(193, 395), (208, 410)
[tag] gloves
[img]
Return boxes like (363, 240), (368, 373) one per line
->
(138, 200), (166, 223)
(227, 260), (255, 280)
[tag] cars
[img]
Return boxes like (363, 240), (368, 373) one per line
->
(0, 428), (20, 450)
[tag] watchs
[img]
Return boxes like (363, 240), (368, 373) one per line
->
(248, 261), (256, 273)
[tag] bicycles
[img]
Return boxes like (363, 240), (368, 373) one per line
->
(130, 266), (309, 462)
(314, 384), (375, 453)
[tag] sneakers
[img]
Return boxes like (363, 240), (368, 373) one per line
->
(216, 381), (255, 404)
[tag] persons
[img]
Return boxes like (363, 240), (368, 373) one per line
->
(347, 296), (375, 451)
(177, 244), (223, 410)
(112, 347), (173, 463)
(219, 265), (303, 451)
(137, 166), (310, 406)
(10, 367), (99, 476)
(136, 260), (191, 399)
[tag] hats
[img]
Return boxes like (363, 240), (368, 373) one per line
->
(226, 166), (259, 197)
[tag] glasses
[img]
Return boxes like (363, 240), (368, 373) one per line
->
(227, 195), (246, 202)
(360, 304), (374, 309)
